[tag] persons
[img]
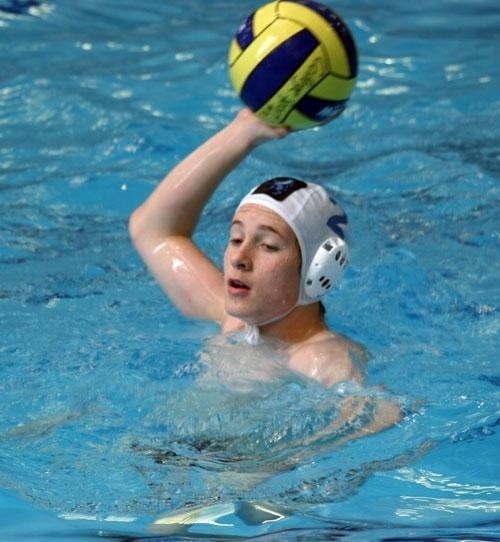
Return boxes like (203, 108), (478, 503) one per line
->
(126, 106), (407, 537)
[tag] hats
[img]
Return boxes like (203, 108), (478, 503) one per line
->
(232, 176), (350, 307)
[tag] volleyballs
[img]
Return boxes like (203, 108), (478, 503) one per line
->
(229, 1), (358, 131)
